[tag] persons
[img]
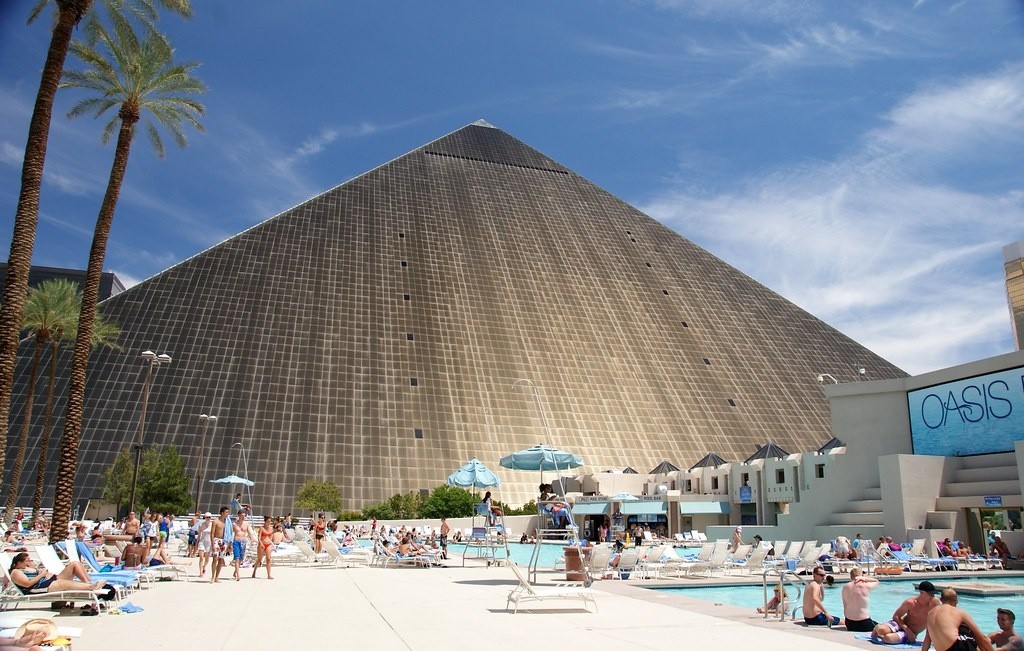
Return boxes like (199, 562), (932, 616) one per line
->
(1, 482), (1023, 650)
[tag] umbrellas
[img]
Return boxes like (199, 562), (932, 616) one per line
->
(446, 455), (501, 528)
(499, 442), (585, 493)
(607, 491), (641, 514)
(207, 474), (257, 516)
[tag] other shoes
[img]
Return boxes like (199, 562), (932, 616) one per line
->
(159, 577), (172, 581)
(80, 603), (105, 616)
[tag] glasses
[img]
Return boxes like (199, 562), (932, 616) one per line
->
(206, 516), (211, 519)
(774, 590), (778, 592)
(817, 573), (826, 577)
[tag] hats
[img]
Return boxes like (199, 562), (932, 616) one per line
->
(919, 581), (939, 594)
(736, 527), (742, 532)
(205, 512), (212, 517)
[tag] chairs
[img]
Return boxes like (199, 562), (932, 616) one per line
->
(0, 511), (1024, 651)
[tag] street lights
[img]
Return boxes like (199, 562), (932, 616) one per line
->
(194, 412), (217, 519)
(128, 348), (172, 516)
